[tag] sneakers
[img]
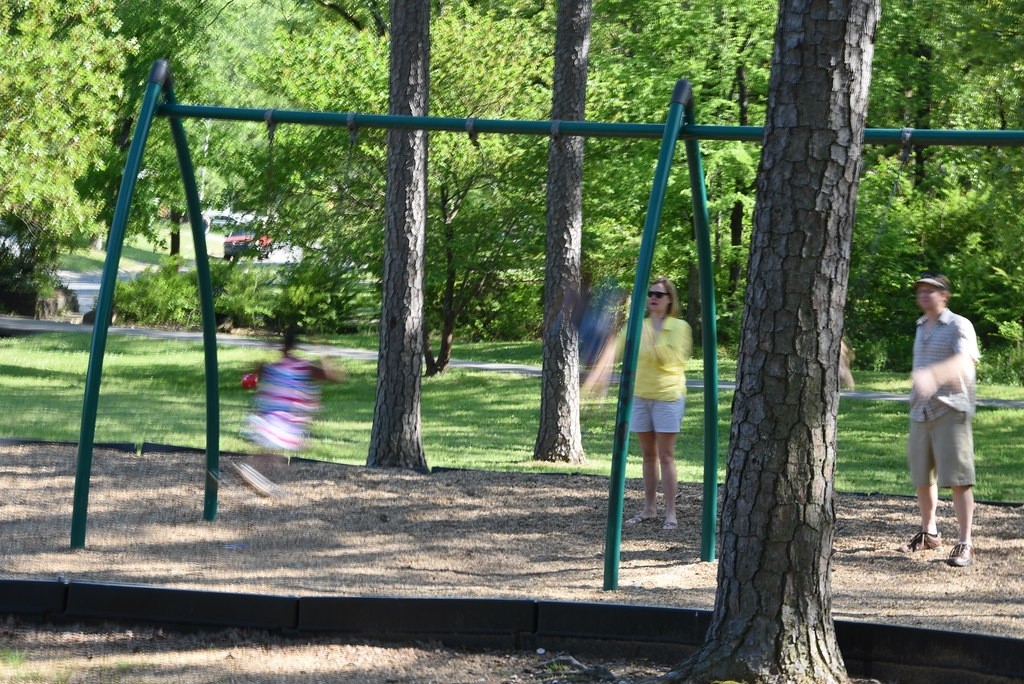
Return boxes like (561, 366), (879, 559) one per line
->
(899, 531), (942, 553)
(948, 544), (974, 566)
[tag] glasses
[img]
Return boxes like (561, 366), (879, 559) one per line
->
(647, 290), (669, 299)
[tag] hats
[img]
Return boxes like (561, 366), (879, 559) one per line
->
(915, 274), (952, 292)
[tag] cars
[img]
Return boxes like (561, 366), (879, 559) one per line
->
(223, 225), (273, 261)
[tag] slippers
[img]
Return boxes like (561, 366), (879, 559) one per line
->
(625, 514), (657, 525)
(663, 520), (678, 530)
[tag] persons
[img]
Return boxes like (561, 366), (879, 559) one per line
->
(898, 278), (979, 567)
(245, 326), (340, 471)
(614, 280), (691, 529)
